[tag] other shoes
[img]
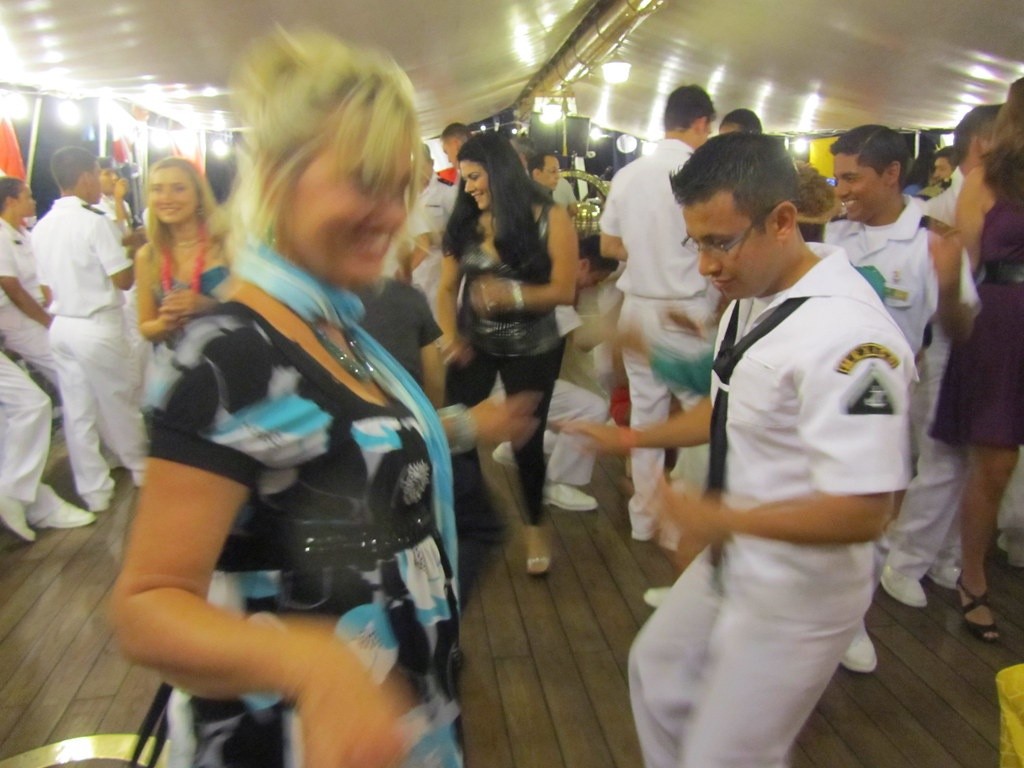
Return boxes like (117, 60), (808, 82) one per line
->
(926, 566), (961, 590)
(491, 442), (519, 469)
(998, 533), (1024, 568)
(630, 522), (678, 553)
(879, 565), (928, 608)
(643, 586), (671, 609)
(542, 483), (598, 511)
(841, 627), (877, 673)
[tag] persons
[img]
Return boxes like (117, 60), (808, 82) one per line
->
(820, 79), (1024, 671)
(133, 158), (236, 365)
(107, 22), (544, 768)
(549, 130), (916, 768)
(383, 122), (618, 574)
(99, 157), (130, 236)
(30, 145), (148, 513)
(718, 106), (762, 135)
(0, 351), (97, 541)
(599, 85), (718, 550)
(0, 178), (53, 393)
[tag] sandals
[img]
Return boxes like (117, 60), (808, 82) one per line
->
(956, 569), (1001, 644)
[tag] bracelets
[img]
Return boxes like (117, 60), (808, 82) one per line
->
(440, 404), (479, 446)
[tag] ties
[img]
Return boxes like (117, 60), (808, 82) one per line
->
(697, 301), (806, 569)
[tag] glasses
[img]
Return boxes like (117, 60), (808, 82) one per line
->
(681, 220), (757, 261)
(547, 168), (562, 174)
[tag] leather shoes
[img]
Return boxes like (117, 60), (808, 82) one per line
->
(24, 484), (96, 530)
(87, 487), (116, 513)
(0, 491), (36, 545)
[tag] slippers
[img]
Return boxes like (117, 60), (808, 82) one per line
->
(525, 555), (554, 579)
